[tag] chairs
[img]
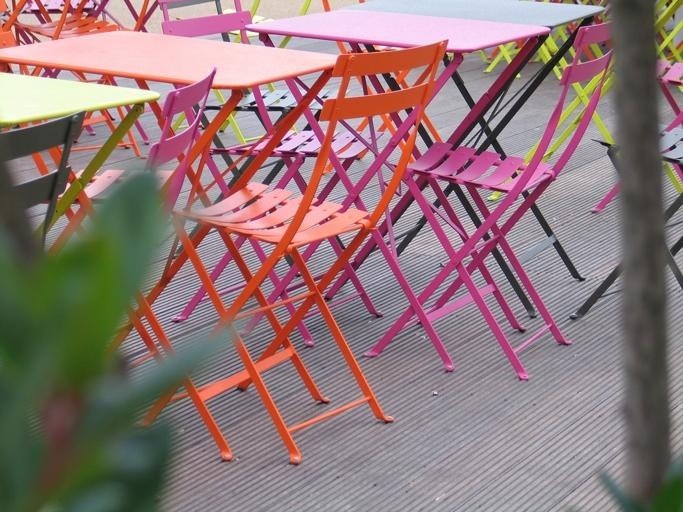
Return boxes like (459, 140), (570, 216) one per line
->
(0, 0), (683, 467)
